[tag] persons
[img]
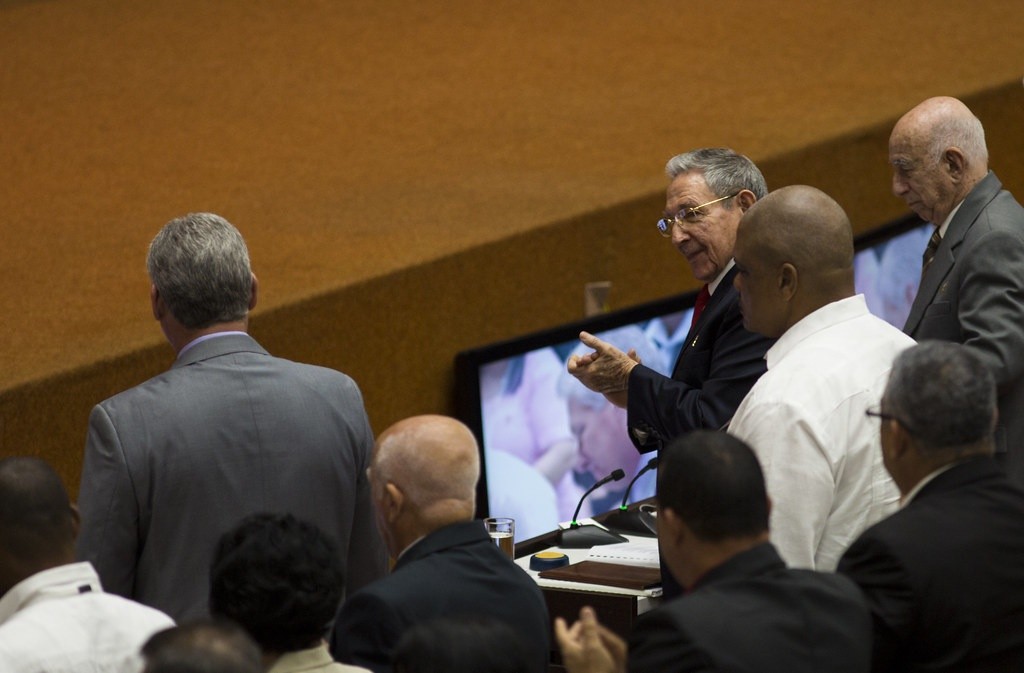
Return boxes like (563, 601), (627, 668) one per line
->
(726, 185), (921, 576)
(328, 414), (555, 673)
(888, 96), (1024, 493)
(567, 148), (788, 612)
(835, 341), (1024, 673)
(0, 457), (178, 673)
(75, 213), (389, 644)
(481, 309), (700, 551)
(208, 509), (373, 673)
(556, 430), (875, 673)
(141, 620), (261, 673)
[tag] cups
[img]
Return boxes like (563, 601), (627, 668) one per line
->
(483, 516), (515, 562)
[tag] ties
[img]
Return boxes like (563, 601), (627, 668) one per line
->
(923, 231), (940, 275)
(689, 284), (709, 334)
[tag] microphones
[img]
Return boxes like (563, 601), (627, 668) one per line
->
(604, 456), (660, 536)
(557, 469), (630, 550)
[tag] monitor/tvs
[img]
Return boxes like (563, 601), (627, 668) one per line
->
(850, 211), (938, 332)
(456, 287), (700, 544)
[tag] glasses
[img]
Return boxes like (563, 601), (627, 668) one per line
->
(656, 191), (739, 239)
(866, 406), (914, 437)
